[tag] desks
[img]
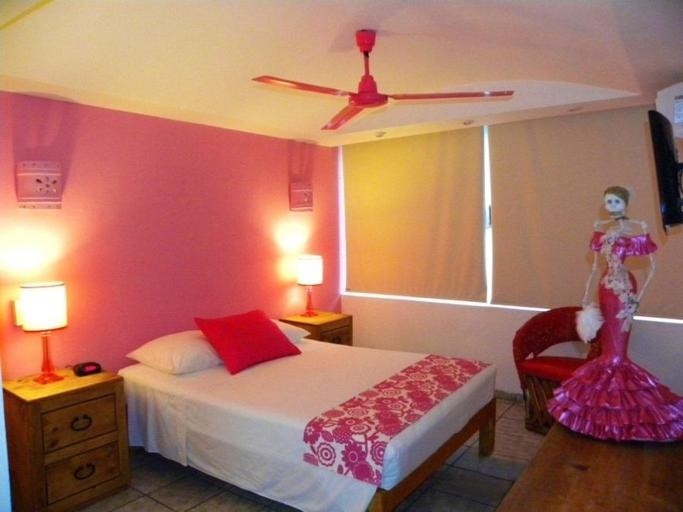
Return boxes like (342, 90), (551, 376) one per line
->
(496, 400), (682, 511)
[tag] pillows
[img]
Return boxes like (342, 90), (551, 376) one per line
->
(124, 309), (311, 376)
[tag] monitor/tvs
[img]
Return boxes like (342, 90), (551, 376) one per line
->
(647, 109), (682, 226)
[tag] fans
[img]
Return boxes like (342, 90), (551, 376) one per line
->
(251, 28), (515, 131)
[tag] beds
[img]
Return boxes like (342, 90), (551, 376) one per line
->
(117, 338), (476, 512)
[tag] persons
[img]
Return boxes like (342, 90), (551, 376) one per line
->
(581, 186), (656, 442)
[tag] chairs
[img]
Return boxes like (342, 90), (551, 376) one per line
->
(513, 306), (602, 435)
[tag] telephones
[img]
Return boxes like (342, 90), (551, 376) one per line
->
(74, 362), (100, 376)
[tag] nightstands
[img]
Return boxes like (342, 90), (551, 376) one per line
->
(3, 370), (132, 512)
(277, 311), (353, 348)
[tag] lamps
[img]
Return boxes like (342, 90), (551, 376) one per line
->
(15, 280), (68, 383)
(296, 254), (323, 317)
